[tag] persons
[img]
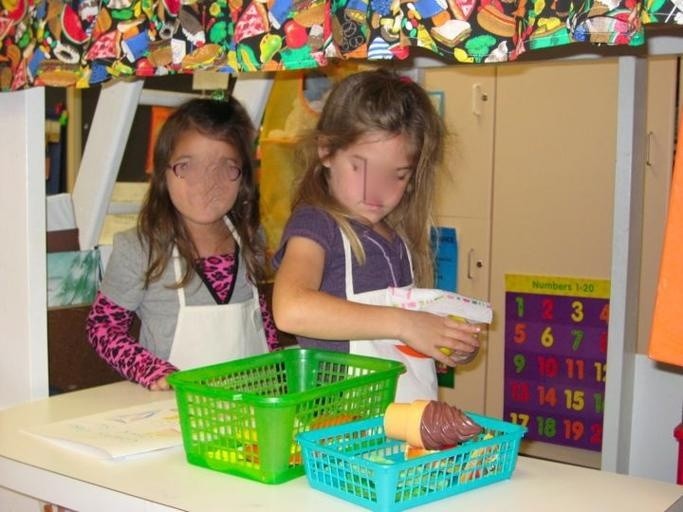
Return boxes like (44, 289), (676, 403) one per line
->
(87, 93), (280, 390)
(268, 67), (487, 406)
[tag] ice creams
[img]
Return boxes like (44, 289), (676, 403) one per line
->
(382, 399), (484, 451)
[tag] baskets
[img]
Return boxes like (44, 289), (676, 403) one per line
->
(294, 405), (528, 512)
(166, 347), (407, 486)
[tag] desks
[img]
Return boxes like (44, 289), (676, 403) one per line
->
(0, 378), (683, 512)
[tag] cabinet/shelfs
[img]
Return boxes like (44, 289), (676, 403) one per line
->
(396, 41), (639, 473)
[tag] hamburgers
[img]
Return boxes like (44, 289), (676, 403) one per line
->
(0, 0), (667, 93)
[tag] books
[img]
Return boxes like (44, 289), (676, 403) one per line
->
(16, 394), (257, 460)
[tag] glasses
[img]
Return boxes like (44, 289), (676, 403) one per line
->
(164, 162), (243, 182)
